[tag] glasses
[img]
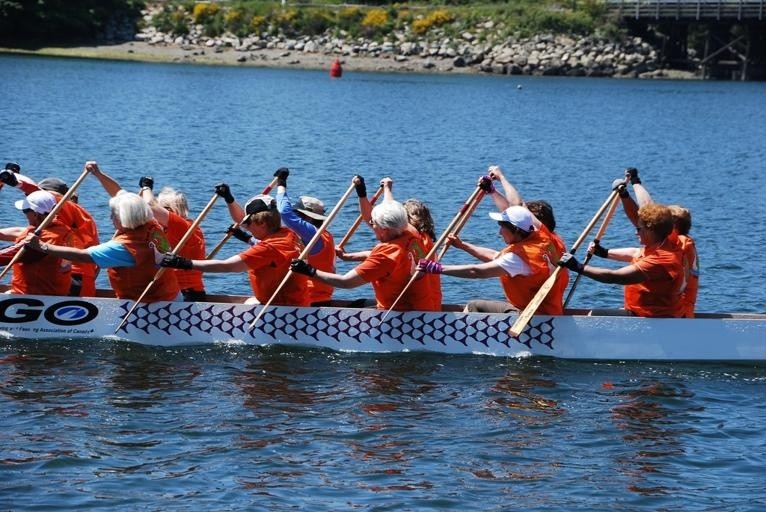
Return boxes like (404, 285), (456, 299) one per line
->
(368, 220), (377, 226)
(635, 226), (646, 232)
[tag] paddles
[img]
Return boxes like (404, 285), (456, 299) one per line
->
(246, 177), (355, 333)
(378, 186), (480, 323)
(1, 169), (90, 279)
(113, 186), (223, 334)
(508, 185), (624, 337)
(562, 172), (632, 309)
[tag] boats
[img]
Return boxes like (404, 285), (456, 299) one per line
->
(0, 281), (765, 363)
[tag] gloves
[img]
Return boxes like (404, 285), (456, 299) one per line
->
(557, 252), (584, 274)
(0, 163), (20, 187)
(625, 168), (641, 185)
(612, 177), (628, 199)
(138, 176), (154, 190)
(273, 168), (288, 187)
(223, 223), (252, 244)
(161, 252), (192, 270)
(215, 183), (233, 204)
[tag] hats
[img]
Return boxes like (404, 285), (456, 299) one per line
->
(240, 194), (276, 225)
(488, 206), (534, 234)
(15, 190), (57, 214)
(295, 195), (328, 220)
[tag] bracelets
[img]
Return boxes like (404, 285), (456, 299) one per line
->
(141, 186), (150, 191)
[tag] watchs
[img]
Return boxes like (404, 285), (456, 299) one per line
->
(41, 243), (49, 254)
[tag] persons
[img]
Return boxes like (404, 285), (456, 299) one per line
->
(0, 169), (79, 294)
(559, 167), (690, 318)
(587, 179), (699, 316)
(0, 167), (110, 297)
(130, 177), (207, 303)
(22, 161), (186, 302)
(331, 177), (443, 311)
(227, 168), (336, 306)
(290, 175), (433, 311)
(449, 167), (570, 307)
(158, 184), (311, 306)
(418, 166), (566, 316)
